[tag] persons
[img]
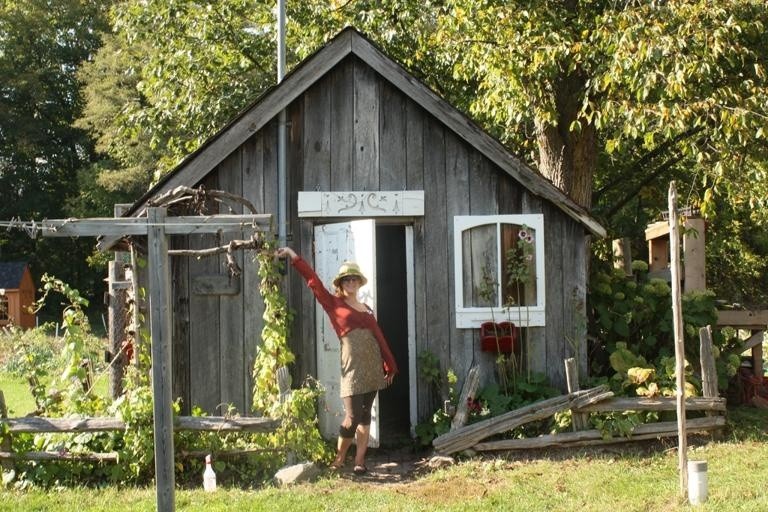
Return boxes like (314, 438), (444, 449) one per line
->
(274, 245), (398, 476)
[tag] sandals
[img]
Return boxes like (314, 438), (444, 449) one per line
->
(329, 461), (368, 475)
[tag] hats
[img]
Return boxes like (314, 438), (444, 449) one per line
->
(332, 261), (367, 288)
